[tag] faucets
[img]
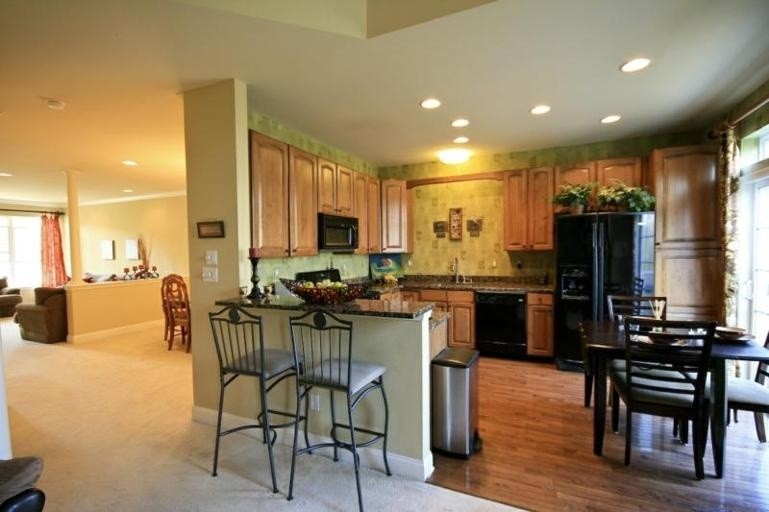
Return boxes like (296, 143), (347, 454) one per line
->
(450, 256), (460, 284)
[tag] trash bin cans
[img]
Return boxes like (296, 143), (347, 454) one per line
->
(430, 347), (481, 460)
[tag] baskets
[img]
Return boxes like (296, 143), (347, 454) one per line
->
(279, 278), (372, 306)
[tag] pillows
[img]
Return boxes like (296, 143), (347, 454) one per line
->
(30, 286), (64, 306)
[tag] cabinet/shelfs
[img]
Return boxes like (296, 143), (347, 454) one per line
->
(351, 171), (381, 256)
(553, 154), (644, 216)
(501, 165), (553, 253)
(358, 285), (556, 363)
(318, 157), (353, 216)
(248, 128), (319, 258)
(377, 177), (406, 254)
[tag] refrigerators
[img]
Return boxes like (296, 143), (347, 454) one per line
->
(551, 210), (656, 372)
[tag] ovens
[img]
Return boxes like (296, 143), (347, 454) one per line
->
(474, 287), (527, 356)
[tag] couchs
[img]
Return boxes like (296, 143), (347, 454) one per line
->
(0, 275), (22, 321)
(12, 286), (67, 345)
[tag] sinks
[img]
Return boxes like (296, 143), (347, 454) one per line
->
(428, 280), (480, 288)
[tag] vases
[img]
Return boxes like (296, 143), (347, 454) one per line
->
(601, 200), (627, 211)
(567, 202), (583, 216)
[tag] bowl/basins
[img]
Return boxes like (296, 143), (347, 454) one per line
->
(624, 315), (663, 330)
(648, 332), (689, 345)
(715, 325), (748, 340)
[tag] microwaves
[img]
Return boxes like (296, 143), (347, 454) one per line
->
(317, 212), (359, 250)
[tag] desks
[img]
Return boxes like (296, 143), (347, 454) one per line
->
(572, 315), (768, 477)
(163, 292), (192, 307)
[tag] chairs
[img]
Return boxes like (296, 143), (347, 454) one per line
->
(159, 272), (188, 344)
(602, 288), (672, 432)
(163, 275), (191, 353)
(282, 307), (393, 512)
(208, 303), (313, 494)
(702, 328), (769, 441)
(609, 307), (717, 482)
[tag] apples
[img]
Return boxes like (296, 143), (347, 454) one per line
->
(297, 279), (342, 288)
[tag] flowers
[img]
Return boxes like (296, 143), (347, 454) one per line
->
(554, 179), (593, 207)
(596, 173), (635, 204)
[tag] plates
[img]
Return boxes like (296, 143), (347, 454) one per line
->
(713, 333), (758, 345)
(628, 335), (689, 349)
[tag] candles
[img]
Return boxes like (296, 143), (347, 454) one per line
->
(247, 245), (261, 259)
(124, 267), (130, 273)
(153, 266), (157, 271)
(139, 265), (146, 269)
(133, 266), (137, 270)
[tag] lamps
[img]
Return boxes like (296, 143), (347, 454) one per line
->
(433, 148), (478, 166)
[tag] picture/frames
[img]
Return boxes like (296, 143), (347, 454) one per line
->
(195, 220), (226, 239)
